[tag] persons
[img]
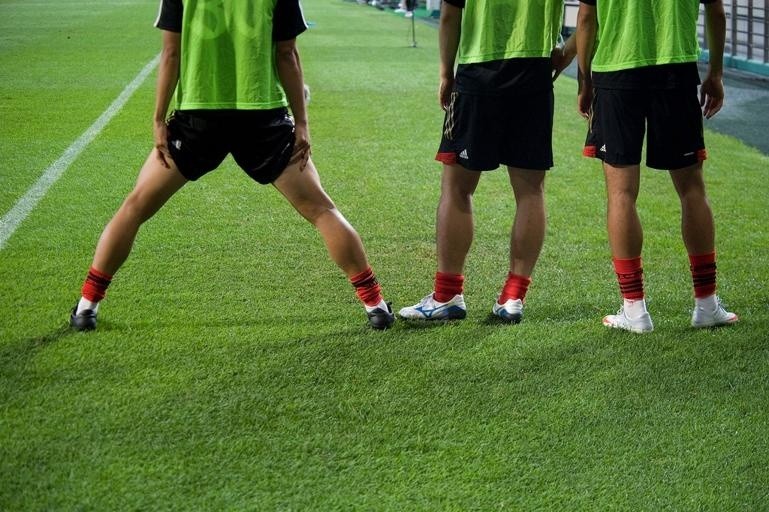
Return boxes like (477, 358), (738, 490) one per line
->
(570, 0), (738, 335)
(396, 1), (587, 325)
(68, 1), (394, 334)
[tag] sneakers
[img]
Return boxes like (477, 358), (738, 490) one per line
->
(366, 302), (395, 330)
(601, 304), (654, 334)
(399, 291), (466, 320)
(71, 302), (97, 332)
(492, 297), (524, 322)
(690, 304), (738, 328)
